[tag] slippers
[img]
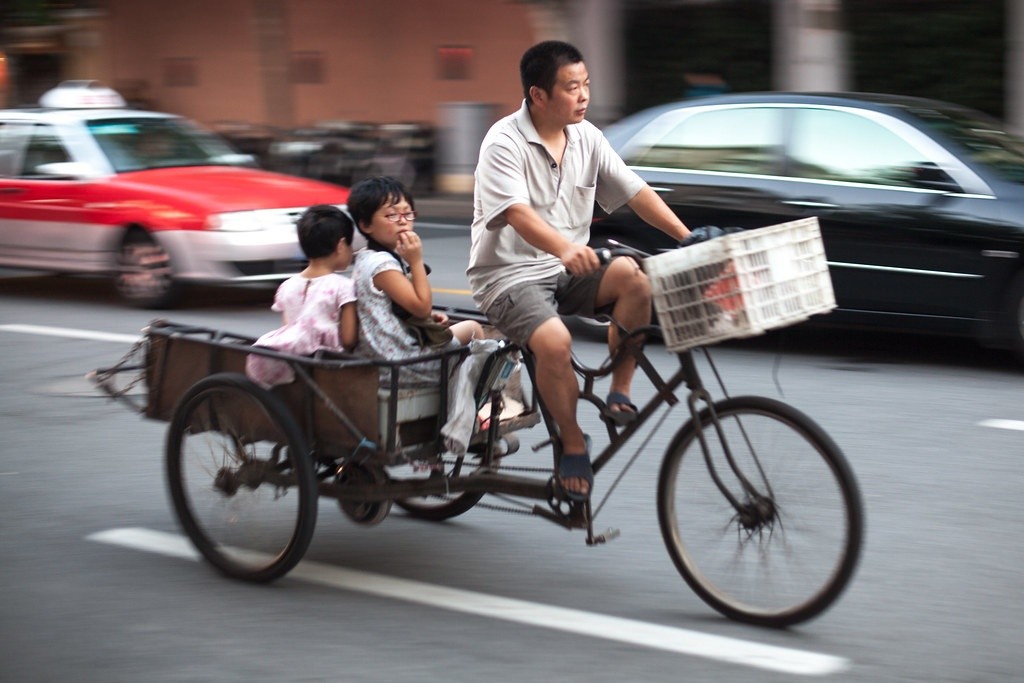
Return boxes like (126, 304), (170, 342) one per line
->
(557, 433), (594, 503)
(597, 392), (638, 427)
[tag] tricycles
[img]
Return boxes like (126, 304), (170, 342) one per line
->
(90, 223), (867, 630)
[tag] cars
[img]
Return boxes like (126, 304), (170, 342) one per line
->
(583, 90), (1024, 373)
(0, 79), (369, 310)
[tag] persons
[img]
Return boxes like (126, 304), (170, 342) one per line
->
(347, 176), (485, 386)
(465, 40), (693, 502)
(245, 203), (357, 392)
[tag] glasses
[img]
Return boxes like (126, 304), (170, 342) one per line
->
(372, 210), (418, 222)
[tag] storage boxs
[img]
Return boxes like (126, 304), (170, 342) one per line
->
(643, 217), (839, 353)
